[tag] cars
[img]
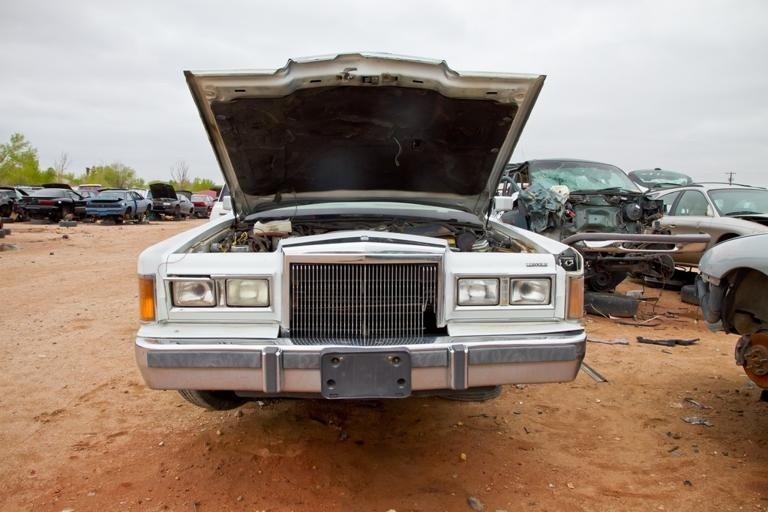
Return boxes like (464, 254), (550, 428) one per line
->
(0, 183), (223, 223)
(132, 52), (587, 410)
